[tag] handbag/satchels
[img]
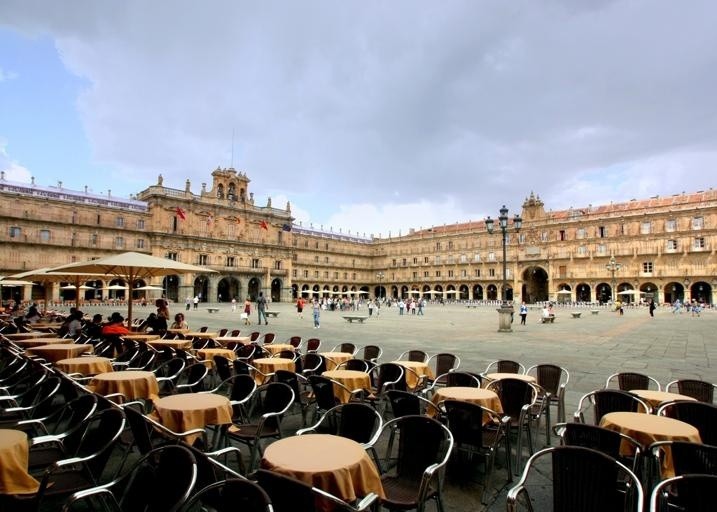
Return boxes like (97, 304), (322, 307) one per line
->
(240, 313), (248, 319)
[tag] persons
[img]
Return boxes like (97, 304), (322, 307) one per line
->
(132, 299), (190, 341)
(519, 300), (528, 326)
(295, 298), (305, 319)
(142, 297), (147, 307)
(232, 297), (237, 312)
(241, 292), (270, 325)
(673, 298), (683, 314)
(319, 296), (427, 316)
(684, 299), (691, 312)
(542, 304), (549, 322)
(548, 305), (555, 322)
(313, 299), (320, 329)
(647, 298), (656, 318)
(219, 293), (221, 303)
(60, 307), (127, 341)
(1, 300), (43, 334)
(185, 295), (199, 310)
(689, 298), (702, 320)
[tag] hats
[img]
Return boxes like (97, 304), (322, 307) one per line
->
(108, 313), (124, 322)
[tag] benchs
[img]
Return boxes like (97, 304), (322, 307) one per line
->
(204, 299), (371, 326)
(538, 309), (599, 324)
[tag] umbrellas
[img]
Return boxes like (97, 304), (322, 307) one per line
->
(423, 288), (442, 300)
(0, 260), (165, 315)
(300, 288), (369, 297)
(443, 288), (465, 300)
(47, 251), (223, 327)
(405, 289), (423, 299)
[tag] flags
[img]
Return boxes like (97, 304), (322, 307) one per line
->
(263, 220), (268, 231)
(206, 212), (215, 230)
(233, 216), (241, 224)
(176, 206), (187, 219)
(283, 223), (292, 231)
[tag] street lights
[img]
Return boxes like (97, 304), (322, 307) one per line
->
(375, 271), (384, 307)
(605, 257), (621, 311)
(484, 204), (521, 332)
